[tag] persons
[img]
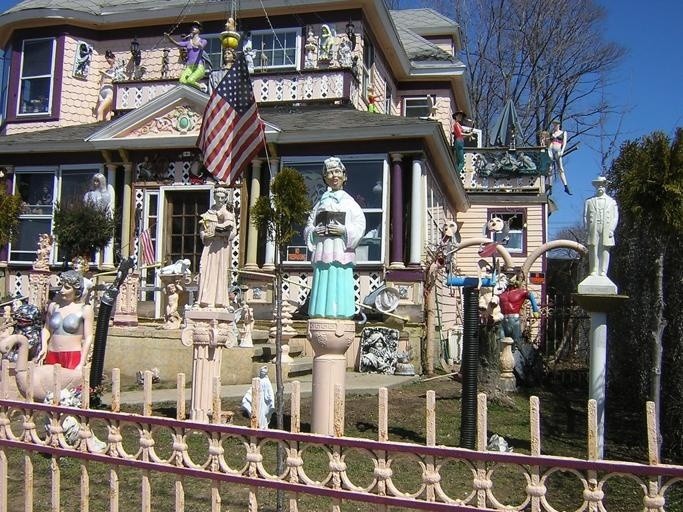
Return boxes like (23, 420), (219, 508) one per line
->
(583, 175), (621, 279)
(547, 120), (573, 197)
(93, 48), (117, 123)
(302, 154), (367, 320)
(359, 330), (395, 374)
(366, 84), (383, 113)
(451, 109), (475, 174)
(207, 47), (238, 97)
(33, 270), (95, 373)
(494, 265), (540, 348)
(162, 20), (208, 93)
(240, 364), (275, 430)
(79, 171), (112, 216)
(319, 24), (331, 63)
(63, 254), (95, 302)
(193, 186), (237, 308)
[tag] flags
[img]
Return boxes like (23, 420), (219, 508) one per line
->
(137, 228), (155, 267)
(195, 50), (265, 189)
